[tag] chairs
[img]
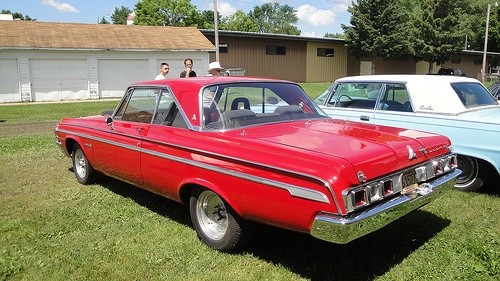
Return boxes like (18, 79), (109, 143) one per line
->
(217, 109), (257, 122)
(273, 104), (304, 116)
(153, 101), (179, 126)
(231, 97), (250, 111)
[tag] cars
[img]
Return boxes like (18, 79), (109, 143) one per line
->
(237, 74), (500, 193)
(486, 81), (500, 101)
(54, 75), (464, 254)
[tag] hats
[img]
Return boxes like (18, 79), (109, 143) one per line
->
(207, 62), (224, 72)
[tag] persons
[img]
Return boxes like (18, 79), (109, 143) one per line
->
(153, 58), (230, 114)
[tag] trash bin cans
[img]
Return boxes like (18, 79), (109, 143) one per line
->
(228, 68), (246, 77)
(208, 70), (228, 76)
